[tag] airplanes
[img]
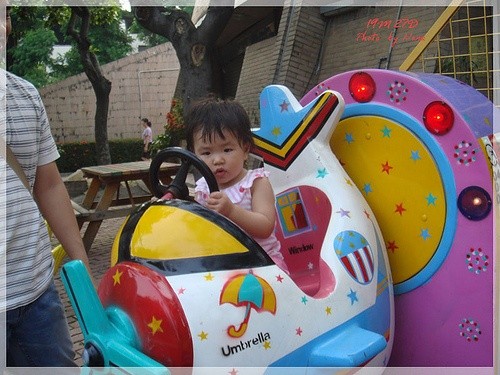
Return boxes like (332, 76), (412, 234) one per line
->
(59, 85), (396, 375)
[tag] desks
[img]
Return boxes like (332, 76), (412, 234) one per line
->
(72, 161), (192, 259)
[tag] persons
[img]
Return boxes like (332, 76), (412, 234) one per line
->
(186, 92), (289, 280)
(0, 0), (97, 374)
(142, 118), (153, 160)
